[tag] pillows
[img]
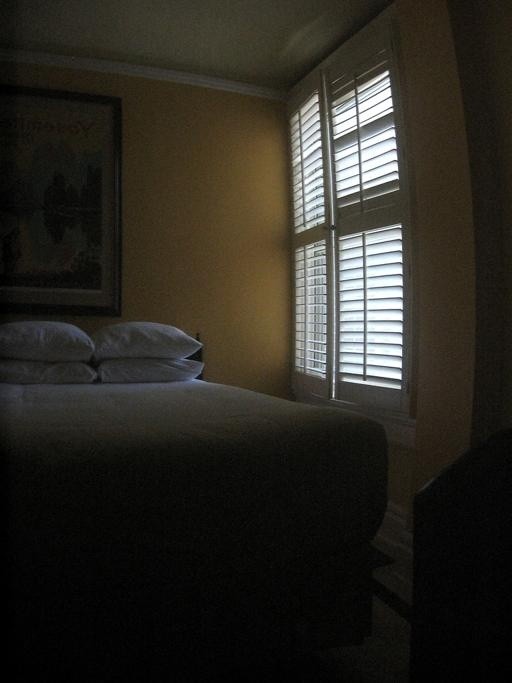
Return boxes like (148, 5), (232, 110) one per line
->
(0, 321), (95, 363)
(0, 357), (98, 383)
(89, 321), (204, 362)
(94, 358), (205, 383)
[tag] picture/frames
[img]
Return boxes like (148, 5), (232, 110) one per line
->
(0, 83), (122, 317)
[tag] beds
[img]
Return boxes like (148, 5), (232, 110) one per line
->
(0, 332), (394, 653)
